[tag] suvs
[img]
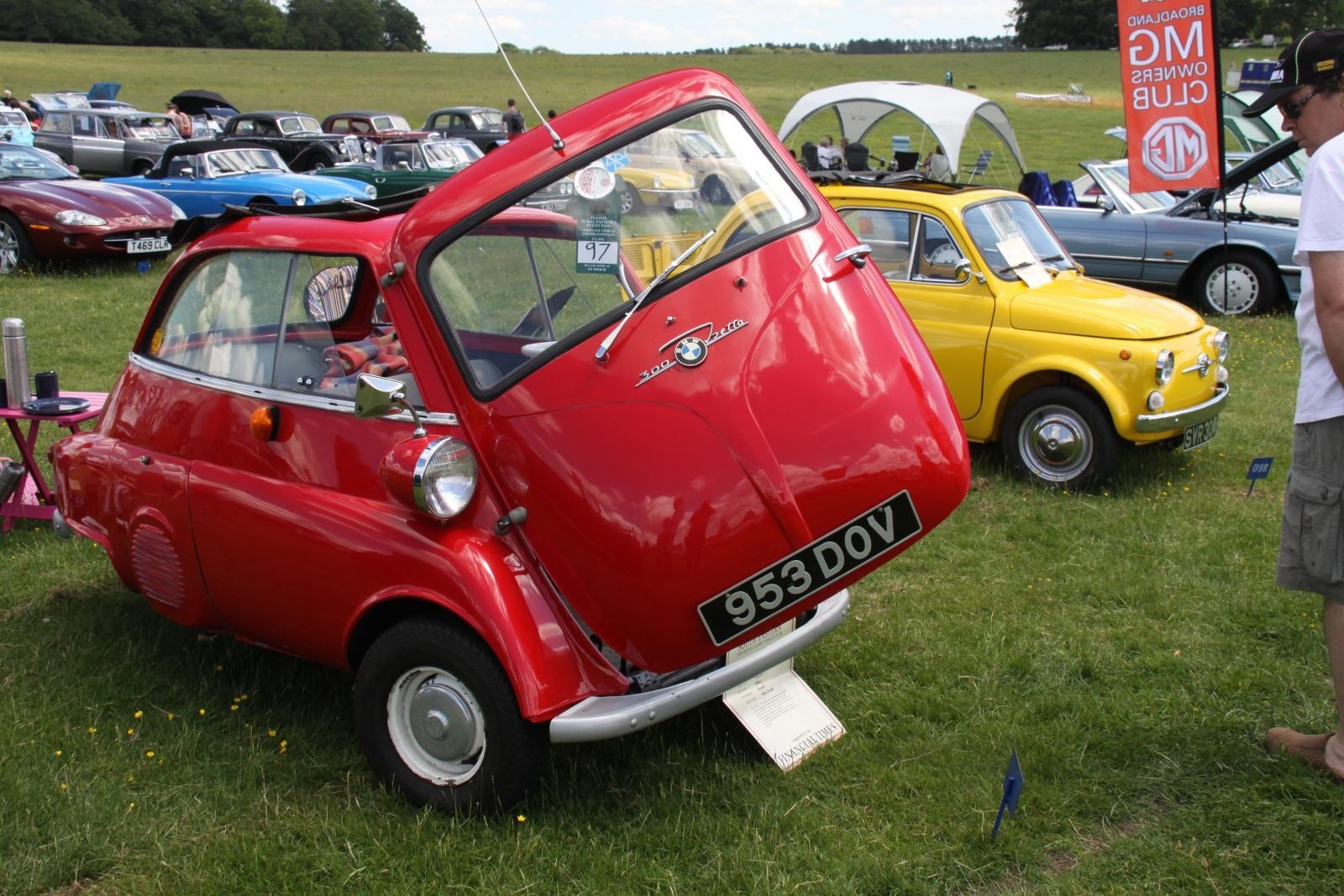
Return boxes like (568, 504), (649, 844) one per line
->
(215, 109), (359, 148)
(412, 105), (524, 146)
(613, 128), (759, 207)
(318, 110), (440, 145)
(1042, 41), (1069, 53)
(33, 109), (189, 182)
(1229, 38), (1253, 49)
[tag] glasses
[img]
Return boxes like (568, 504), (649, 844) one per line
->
(1279, 82), (1333, 118)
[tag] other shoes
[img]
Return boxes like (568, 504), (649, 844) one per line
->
(1263, 728), (1342, 781)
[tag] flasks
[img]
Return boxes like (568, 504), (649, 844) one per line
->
(2, 318), (32, 411)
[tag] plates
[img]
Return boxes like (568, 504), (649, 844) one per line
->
(23, 397), (89, 415)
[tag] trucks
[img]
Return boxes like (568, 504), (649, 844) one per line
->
(1261, 35), (1280, 48)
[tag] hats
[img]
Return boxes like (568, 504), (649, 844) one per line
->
(1240, 27), (1343, 118)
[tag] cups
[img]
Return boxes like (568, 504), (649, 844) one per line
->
(34, 370), (59, 400)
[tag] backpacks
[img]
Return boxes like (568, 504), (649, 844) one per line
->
(513, 110), (525, 130)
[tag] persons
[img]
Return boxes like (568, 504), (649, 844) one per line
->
(815, 134), (846, 171)
(173, 105), (193, 138)
(839, 138), (850, 167)
(1240, 29), (1343, 783)
(943, 69), (955, 88)
(546, 110), (557, 122)
(500, 99), (527, 141)
(1, 89), (21, 109)
(160, 102), (183, 138)
(921, 145), (952, 183)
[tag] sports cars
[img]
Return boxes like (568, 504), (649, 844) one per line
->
(479, 140), (577, 214)
(566, 163), (698, 216)
(295, 138), (485, 210)
(859, 156), (1308, 320)
(98, 137), (379, 239)
(1, 141), (191, 289)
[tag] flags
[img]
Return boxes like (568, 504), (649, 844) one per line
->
(1117, 0), (1226, 196)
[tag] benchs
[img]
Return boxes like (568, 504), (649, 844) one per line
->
(321, 358), (502, 407)
(164, 340), (331, 394)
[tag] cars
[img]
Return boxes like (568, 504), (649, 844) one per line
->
(39, 62), (972, 834)
(652, 180), (1237, 492)
(1060, 159), (1307, 222)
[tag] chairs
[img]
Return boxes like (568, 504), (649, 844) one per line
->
(1019, 172), (1058, 205)
(956, 148), (993, 184)
(801, 142), (821, 170)
(921, 153), (952, 183)
(895, 151), (919, 173)
(1053, 179), (1081, 208)
(845, 143), (872, 171)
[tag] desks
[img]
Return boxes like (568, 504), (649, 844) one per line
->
(1, 390), (111, 534)
